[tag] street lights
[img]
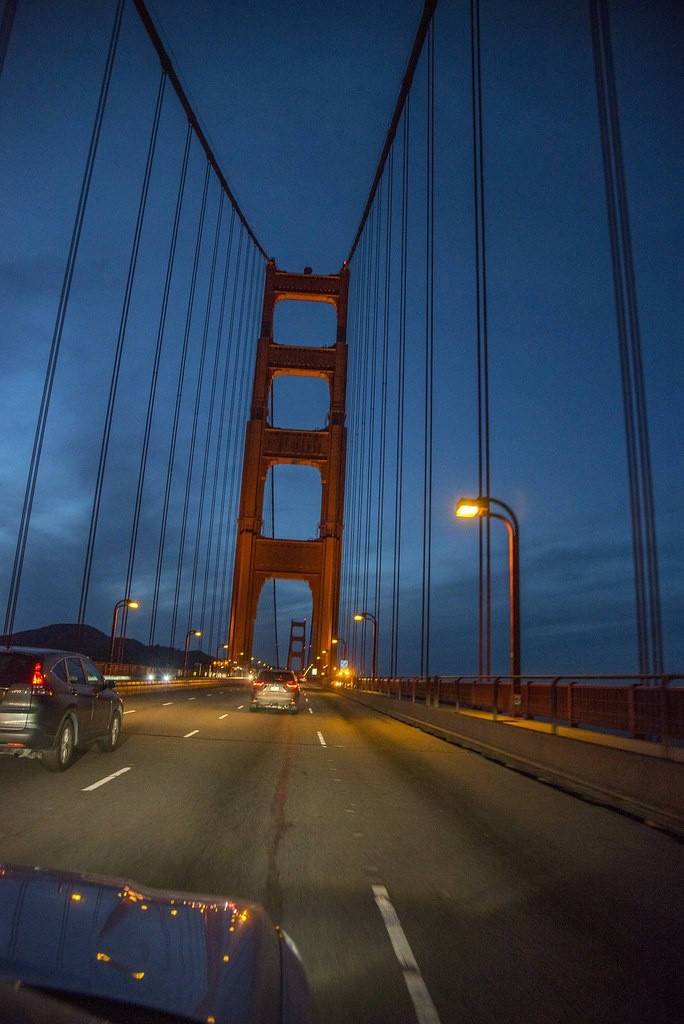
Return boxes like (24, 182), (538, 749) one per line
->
(215, 643), (228, 678)
(354, 613), (380, 679)
(330, 638), (347, 661)
(108, 599), (139, 676)
(183, 630), (201, 677)
(455, 498), (524, 717)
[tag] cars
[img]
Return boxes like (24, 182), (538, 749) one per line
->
(0, 646), (124, 773)
(0, 865), (316, 1024)
(250, 669), (302, 714)
